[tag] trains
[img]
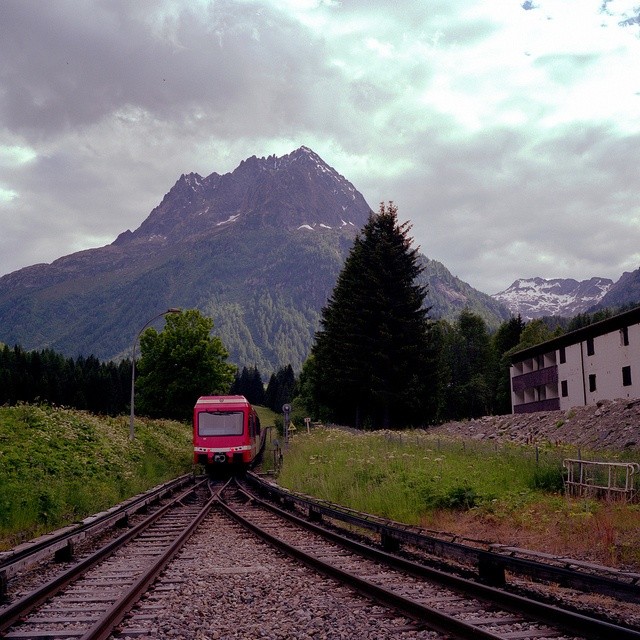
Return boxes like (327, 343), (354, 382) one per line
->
(193, 394), (267, 473)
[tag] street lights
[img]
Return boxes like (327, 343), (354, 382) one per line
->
(130, 307), (182, 443)
